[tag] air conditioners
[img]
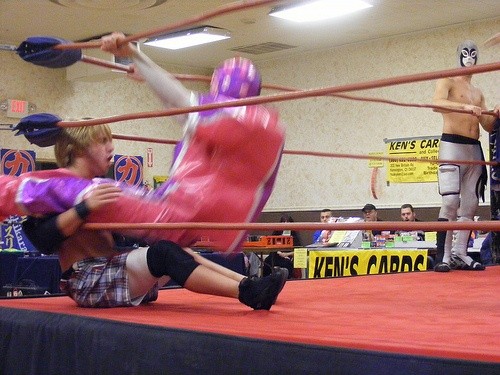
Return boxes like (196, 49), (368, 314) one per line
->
(67, 40), (139, 82)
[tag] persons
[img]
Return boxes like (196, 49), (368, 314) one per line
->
(431, 40), (500, 272)
(391, 204), (425, 241)
(313, 209), (332, 243)
(0, 31), (285, 250)
(362, 204), (395, 235)
(21, 118), (288, 311)
(265, 215), (299, 279)
(454, 198), (479, 246)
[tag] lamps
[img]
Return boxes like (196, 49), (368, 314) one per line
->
(143, 27), (231, 50)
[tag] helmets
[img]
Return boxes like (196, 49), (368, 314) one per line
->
(208, 56), (263, 98)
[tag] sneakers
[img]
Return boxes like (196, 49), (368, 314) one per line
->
(238, 267), (290, 311)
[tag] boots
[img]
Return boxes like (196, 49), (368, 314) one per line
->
(434, 217), (454, 273)
(450, 215), (486, 271)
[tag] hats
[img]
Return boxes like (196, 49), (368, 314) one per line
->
(361, 204), (377, 211)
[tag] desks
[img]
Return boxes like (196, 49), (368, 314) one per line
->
(0, 246), (482, 298)
